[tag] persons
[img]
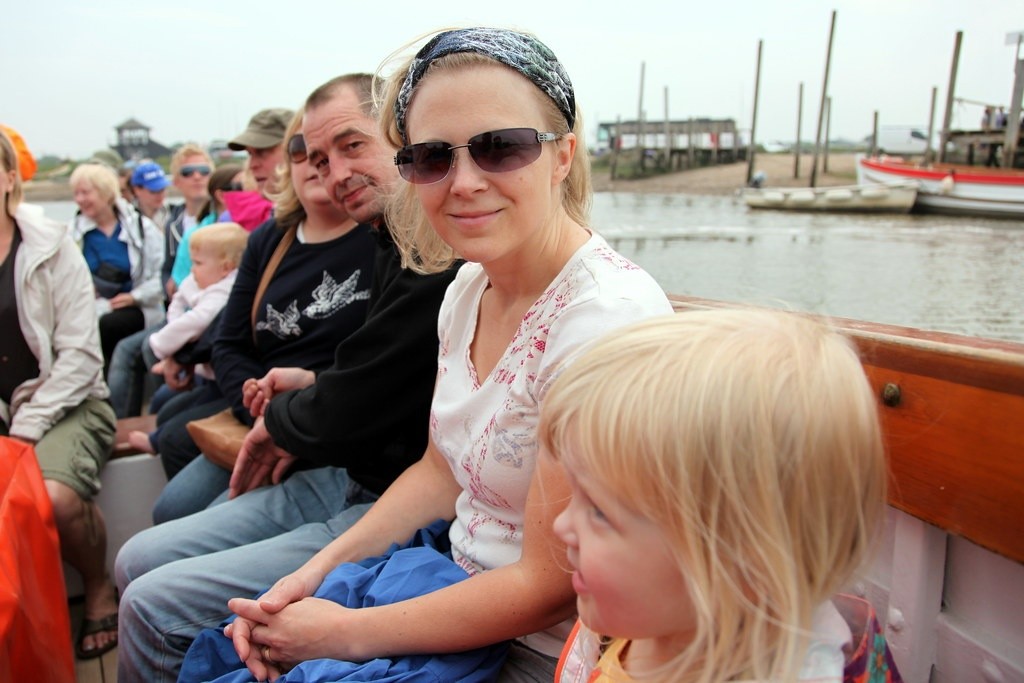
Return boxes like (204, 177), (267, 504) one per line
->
(532, 306), (907, 683)
(112, 72), (466, 683)
(0, 103), (375, 660)
(180, 23), (677, 682)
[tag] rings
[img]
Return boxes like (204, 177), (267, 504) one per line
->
(265, 647), (272, 662)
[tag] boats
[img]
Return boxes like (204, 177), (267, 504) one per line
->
(734, 179), (925, 215)
(950, 128), (1006, 146)
(855, 156), (1024, 223)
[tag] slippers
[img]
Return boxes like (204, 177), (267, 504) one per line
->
(75, 589), (119, 659)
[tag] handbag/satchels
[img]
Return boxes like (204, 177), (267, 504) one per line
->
(186, 407), (252, 470)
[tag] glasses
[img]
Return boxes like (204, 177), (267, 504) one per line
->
(181, 164), (209, 177)
(393, 127), (562, 185)
(288, 135), (307, 163)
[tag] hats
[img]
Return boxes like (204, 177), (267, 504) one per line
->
(131, 162), (172, 192)
(227, 107), (295, 151)
